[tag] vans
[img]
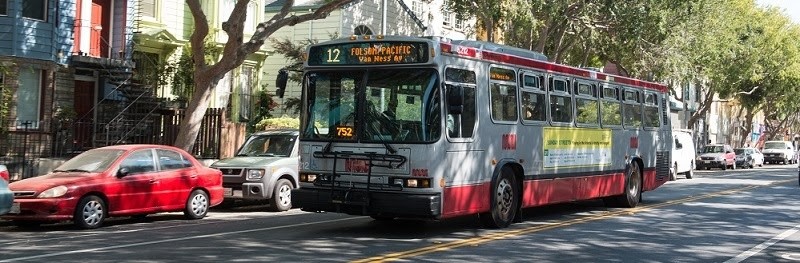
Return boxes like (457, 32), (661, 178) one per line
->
(762, 140), (794, 165)
(670, 129), (697, 181)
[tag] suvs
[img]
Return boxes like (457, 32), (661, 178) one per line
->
(209, 129), (301, 211)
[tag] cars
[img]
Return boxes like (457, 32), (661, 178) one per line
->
(9, 144), (225, 229)
(0, 164), (15, 217)
(746, 148), (764, 167)
(695, 143), (737, 170)
(733, 148), (754, 169)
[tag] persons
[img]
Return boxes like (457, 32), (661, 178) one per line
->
(427, 101), (455, 137)
(274, 138), (286, 154)
(525, 107), (540, 120)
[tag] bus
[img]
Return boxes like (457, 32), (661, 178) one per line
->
(276, 35), (673, 227)
